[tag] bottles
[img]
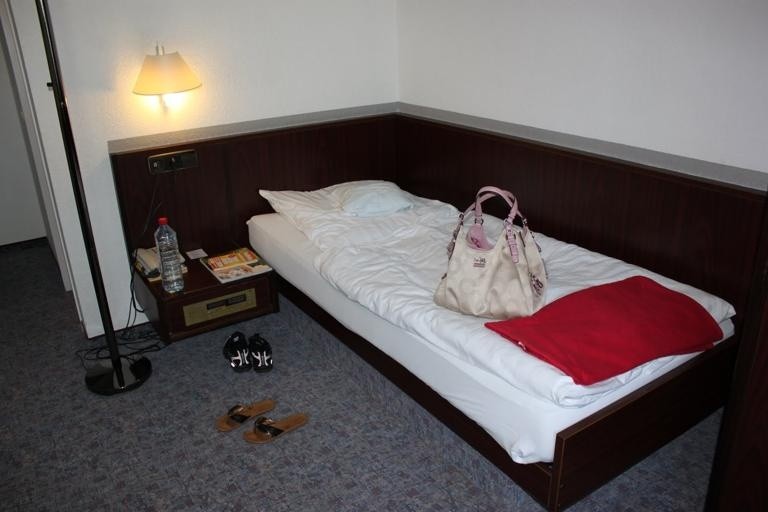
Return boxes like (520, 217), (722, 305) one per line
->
(154, 217), (185, 293)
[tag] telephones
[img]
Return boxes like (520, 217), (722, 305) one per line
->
(132, 246), (185, 272)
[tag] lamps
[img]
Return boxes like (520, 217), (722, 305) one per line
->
(131, 40), (202, 112)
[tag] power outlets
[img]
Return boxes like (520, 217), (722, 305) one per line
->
(147, 149), (199, 176)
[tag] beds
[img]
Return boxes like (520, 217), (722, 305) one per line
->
(246, 179), (737, 512)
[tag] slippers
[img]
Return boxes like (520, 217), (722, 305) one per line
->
(217, 399), (275, 433)
(243, 413), (308, 444)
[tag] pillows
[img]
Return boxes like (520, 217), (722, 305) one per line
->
(330, 181), (413, 213)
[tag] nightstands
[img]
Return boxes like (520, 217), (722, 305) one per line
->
(131, 237), (280, 345)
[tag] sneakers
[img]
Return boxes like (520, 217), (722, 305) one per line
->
(223, 331), (252, 372)
(248, 333), (273, 373)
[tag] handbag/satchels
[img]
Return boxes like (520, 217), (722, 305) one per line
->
(432, 186), (549, 320)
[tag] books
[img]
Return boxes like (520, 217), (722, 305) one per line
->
(201, 248), (272, 285)
(133, 244), (188, 282)
